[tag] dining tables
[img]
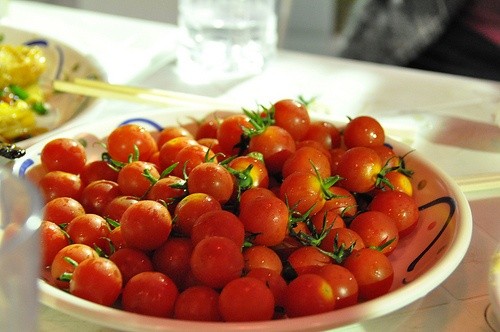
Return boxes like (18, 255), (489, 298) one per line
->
(0, 0), (500, 332)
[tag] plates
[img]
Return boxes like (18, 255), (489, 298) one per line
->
(0, 23), (108, 149)
(25, 117), (473, 332)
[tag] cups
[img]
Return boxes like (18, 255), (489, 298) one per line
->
(176, 0), (278, 79)
(0, 168), (45, 332)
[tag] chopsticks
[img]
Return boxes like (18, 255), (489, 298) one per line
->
(454, 174), (500, 192)
(53, 78), (231, 110)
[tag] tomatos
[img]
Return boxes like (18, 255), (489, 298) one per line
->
(0, 98), (421, 323)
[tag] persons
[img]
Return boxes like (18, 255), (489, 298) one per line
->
(326, 0), (500, 81)
